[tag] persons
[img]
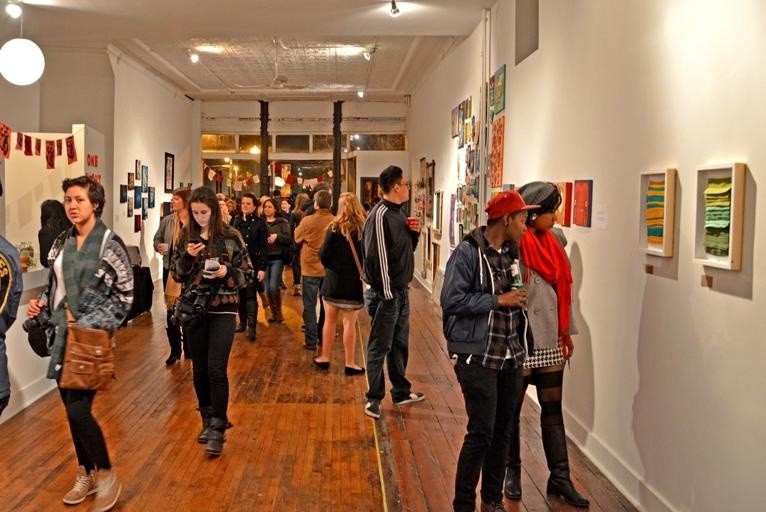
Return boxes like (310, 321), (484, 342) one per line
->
(168, 185), (254, 458)
(362, 163), (427, 419)
(34, 197), (73, 268)
(440, 187), (541, 512)
(0, 178), (22, 432)
(513, 180), (592, 510)
(26, 175), (134, 511)
(153, 181), (368, 377)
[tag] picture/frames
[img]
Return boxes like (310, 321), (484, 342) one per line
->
(163, 151), (175, 194)
(635, 167), (678, 259)
(360, 155), (463, 285)
(691, 161), (750, 271)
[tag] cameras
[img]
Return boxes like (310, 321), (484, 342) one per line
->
(189, 284), (213, 314)
(22, 304), (51, 332)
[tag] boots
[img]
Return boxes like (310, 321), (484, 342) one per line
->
(245, 300), (259, 342)
(502, 416), (523, 501)
(540, 413), (591, 508)
(235, 303), (248, 333)
(196, 407), (228, 457)
(182, 326), (193, 360)
(164, 326), (182, 366)
(258, 288), (285, 323)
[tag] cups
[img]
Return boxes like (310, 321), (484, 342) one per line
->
(159, 244), (168, 255)
(509, 263), (523, 290)
(408, 216), (416, 228)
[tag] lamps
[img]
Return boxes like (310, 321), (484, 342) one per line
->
(184, 0), (401, 99)
(0, 0), (45, 87)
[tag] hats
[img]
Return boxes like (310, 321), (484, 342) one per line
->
(484, 188), (543, 221)
(516, 180), (557, 206)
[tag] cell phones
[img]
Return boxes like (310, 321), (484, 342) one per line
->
(189, 238), (200, 244)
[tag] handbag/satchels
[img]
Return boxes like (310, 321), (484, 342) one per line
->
(171, 297), (201, 328)
(25, 308), (56, 358)
(360, 278), (373, 308)
(57, 321), (119, 392)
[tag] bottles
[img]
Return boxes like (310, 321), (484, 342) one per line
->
(16, 241), (35, 271)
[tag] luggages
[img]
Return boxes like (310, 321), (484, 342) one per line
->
(115, 263), (155, 332)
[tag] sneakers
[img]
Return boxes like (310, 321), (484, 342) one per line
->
(480, 498), (507, 512)
(291, 283), (303, 297)
(364, 401), (382, 420)
(302, 338), (323, 351)
(391, 392), (426, 406)
(61, 468), (101, 505)
(313, 353), (330, 370)
(91, 468), (123, 512)
(300, 324), (309, 333)
(345, 366), (365, 376)
